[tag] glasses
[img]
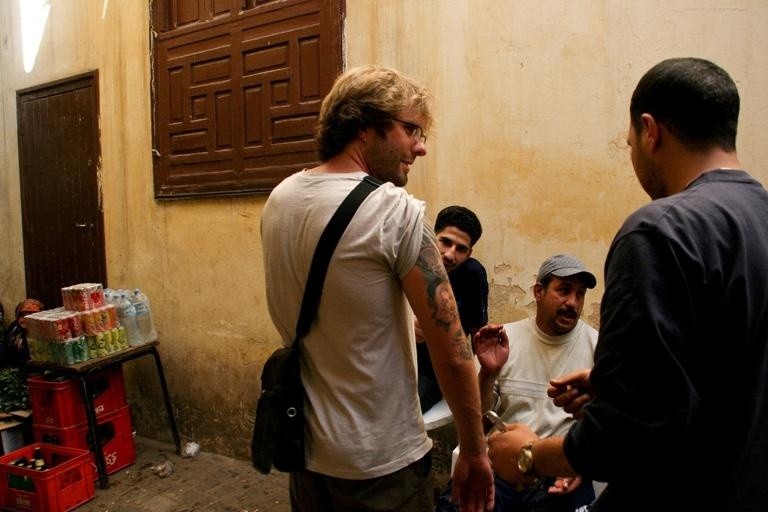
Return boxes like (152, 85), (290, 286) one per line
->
(387, 115), (427, 145)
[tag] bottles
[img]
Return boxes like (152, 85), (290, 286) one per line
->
(103, 288), (156, 347)
(8, 447), (47, 490)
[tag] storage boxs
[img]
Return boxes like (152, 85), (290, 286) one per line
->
(24, 356), (129, 428)
(1, 441), (97, 512)
(30, 403), (137, 484)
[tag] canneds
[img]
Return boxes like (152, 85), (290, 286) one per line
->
(24, 282), (129, 364)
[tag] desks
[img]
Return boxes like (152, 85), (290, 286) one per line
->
(23, 337), (182, 491)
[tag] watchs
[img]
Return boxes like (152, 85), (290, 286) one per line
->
(516, 441), (544, 490)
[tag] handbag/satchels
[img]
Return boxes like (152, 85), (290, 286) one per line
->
(250, 346), (306, 475)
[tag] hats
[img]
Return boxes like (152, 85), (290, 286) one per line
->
(536, 252), (597, 289)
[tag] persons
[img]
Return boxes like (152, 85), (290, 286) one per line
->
(261, 63), (495, 512)
(435, 253), (608, 512)
(5, 298), (44, 365)
(413, 205), (490, 417)
(487, 56), (768, 512)
